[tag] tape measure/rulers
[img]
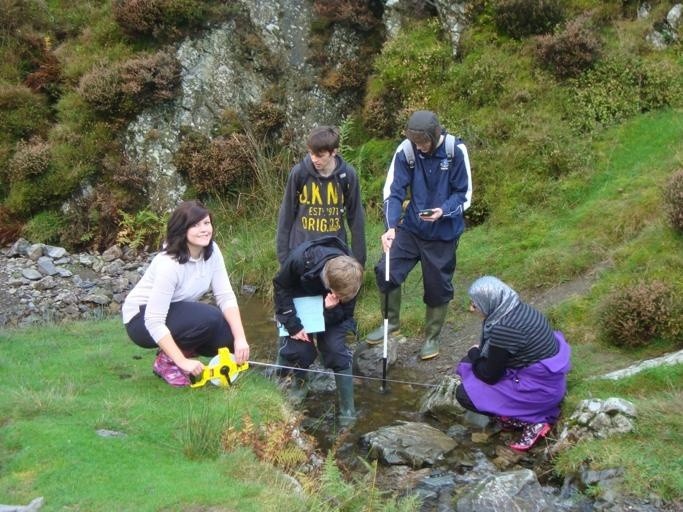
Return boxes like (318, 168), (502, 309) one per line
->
(188, 346), (436, 387)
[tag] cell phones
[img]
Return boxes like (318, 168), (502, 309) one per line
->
(420, 209), (433, 217)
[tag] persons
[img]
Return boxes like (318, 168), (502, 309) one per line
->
(270, 236), (364, 426)
(276, 126), (367, 269)
(456, 275), (572, 450)
(364, 109), (472, 359)
(121, 200), (251, 387)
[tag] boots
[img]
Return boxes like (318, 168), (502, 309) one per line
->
(288, 372), (310, 410)
(153, 349), (192, 388)
(421, 302), (449, 359)
(501, 414), (552, 450)
(365, 284), (401, 345)
(333, 367), (357, 432)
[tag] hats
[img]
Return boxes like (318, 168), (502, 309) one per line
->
(406, 109), (442, 154)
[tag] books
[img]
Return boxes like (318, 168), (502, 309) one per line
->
(277, 294), (326, 337)
